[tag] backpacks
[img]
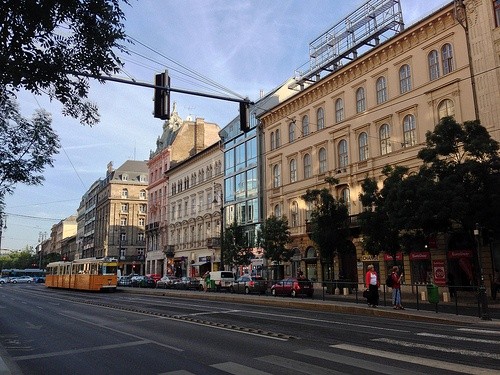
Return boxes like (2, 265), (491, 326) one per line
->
(386, 273), (396, 287)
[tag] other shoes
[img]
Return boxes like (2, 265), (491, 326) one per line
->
(393, 306), (404, 310)
(372, 305), (376, 308)
(367, 303), (371, 307)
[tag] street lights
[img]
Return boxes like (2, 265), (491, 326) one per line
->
(212, 182), (224, 271)
(118, 232), (126, 269)
(473, 222), (491, 321)
(38, 231), (46, 269)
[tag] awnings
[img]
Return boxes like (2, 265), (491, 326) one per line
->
(191, 261), (208, 266)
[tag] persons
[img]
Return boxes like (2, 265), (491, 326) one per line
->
(492, 269), (500, 301)
(390, 266), (406, 310)
(366, 265), (379, 308)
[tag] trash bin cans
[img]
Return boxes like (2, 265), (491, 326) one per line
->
(426, 284), (439, 304)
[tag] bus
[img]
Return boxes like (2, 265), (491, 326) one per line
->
(45, 257), (118, 293)
(1, 268), (46, 283)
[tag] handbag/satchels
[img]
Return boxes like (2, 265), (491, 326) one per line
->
(362, 288), (372, 298)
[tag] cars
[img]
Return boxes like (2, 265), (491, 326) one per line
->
(10, 276), (33, 284)
(173, 276), (200, 290)
(229, 275), (268, 294)
(117, 273), (162, 288)
(156, 275), (180, 289)
(0, 277), (6, 284)
(271, 277), (314, 298)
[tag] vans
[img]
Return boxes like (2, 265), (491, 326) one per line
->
(198, 271), (235, 292)
